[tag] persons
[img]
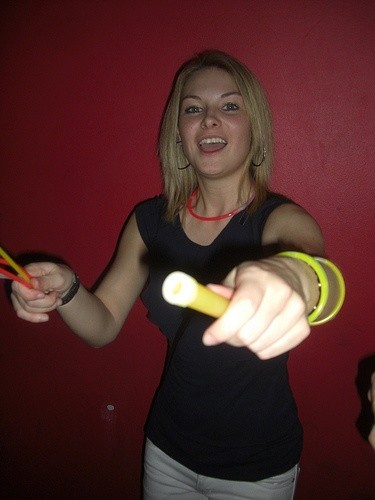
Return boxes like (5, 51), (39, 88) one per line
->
(8, 50), (326, 500)
(356, 354), (375, 449)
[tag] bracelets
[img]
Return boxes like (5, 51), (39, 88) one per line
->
(271, 251), (346, 327)
(61, 271), (80, 305)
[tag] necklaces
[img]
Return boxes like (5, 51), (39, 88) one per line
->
(187, 179), (258, 222)
